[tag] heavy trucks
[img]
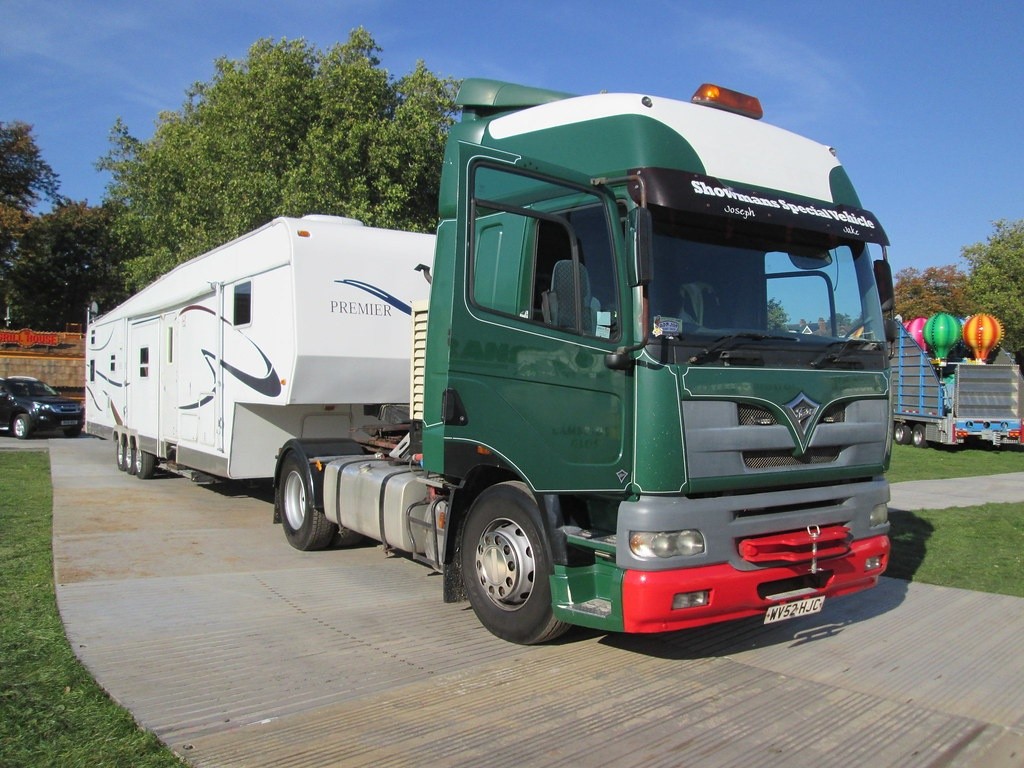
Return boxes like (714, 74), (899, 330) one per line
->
(80, 78), (899, 649)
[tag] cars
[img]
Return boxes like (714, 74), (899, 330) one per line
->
(0, 375), (85, 439)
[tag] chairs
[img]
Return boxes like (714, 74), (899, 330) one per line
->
(539, 259), (601, 336)
